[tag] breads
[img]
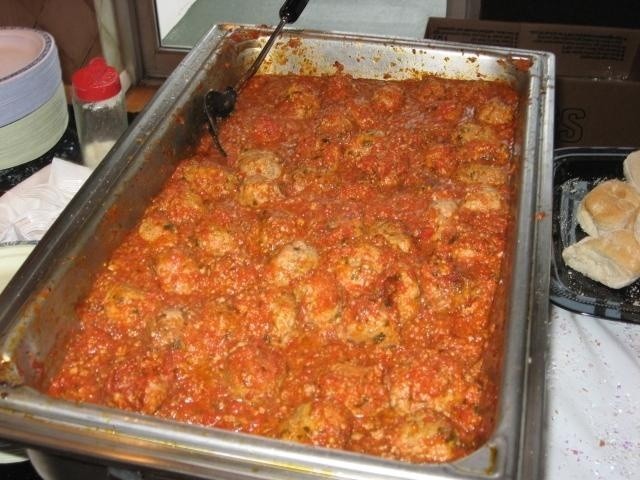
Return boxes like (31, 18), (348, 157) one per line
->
(560, 150), (640, 291)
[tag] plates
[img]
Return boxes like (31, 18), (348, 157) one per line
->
(0, 25), (69, 172)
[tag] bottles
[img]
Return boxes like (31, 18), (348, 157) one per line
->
(71, 58), (128, 171)
